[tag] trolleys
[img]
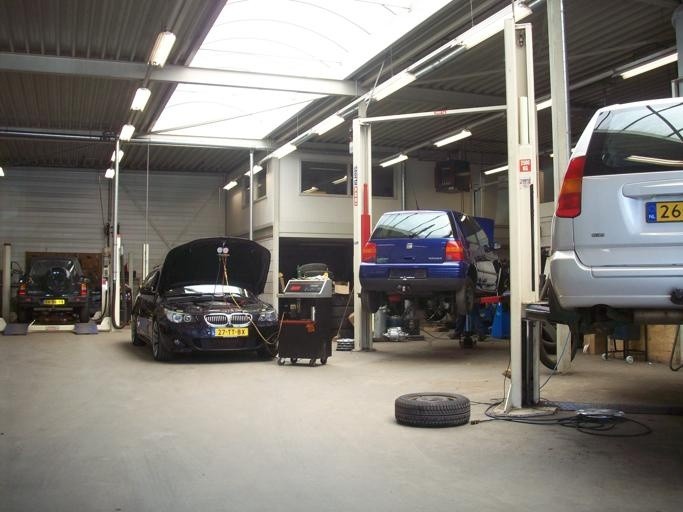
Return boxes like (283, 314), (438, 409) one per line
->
(274, 276), (337, 367)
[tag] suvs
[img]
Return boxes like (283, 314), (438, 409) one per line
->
(8, 254), (101, 323)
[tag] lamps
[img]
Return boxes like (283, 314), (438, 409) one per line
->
(453, 0), (534, 51)
(145, 30), (177, 69)
(379, 152), (409, 169)
(431, 129), (472, 149)
(612, 50), (678, 81)
(128, 87), (152, 113)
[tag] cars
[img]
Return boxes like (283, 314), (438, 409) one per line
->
(125, 236), (281, 362)
(546, 95), (682, 333)
(359, 210), (504, 318)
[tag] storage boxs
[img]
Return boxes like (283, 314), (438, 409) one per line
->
(582, 334), (604, 354)
(332, 279), (350, 295)
